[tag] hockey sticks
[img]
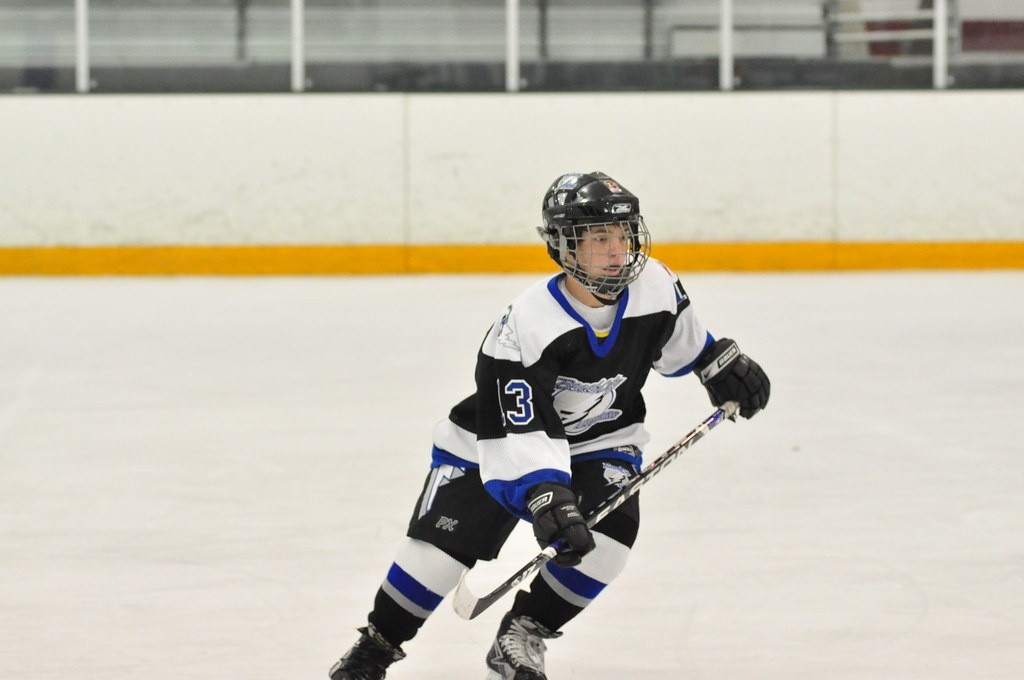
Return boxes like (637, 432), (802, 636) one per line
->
(450, 400), (738, 623)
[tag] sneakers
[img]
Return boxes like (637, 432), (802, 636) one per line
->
(485, 588), (562, 680)
(328, 627), (406, 680)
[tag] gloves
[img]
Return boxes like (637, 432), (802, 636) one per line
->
(693, 337), (771, 423)
(527, 483), (595, 569)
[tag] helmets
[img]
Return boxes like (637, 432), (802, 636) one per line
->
(542, 171), (641, 293)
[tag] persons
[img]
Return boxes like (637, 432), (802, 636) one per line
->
(329, 172), (770, 680)
